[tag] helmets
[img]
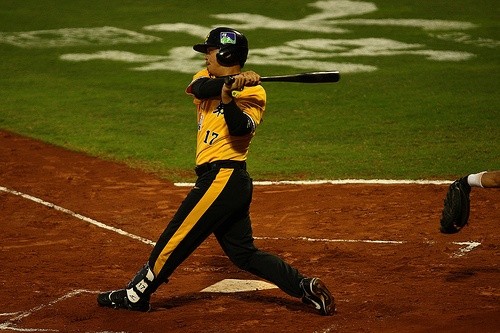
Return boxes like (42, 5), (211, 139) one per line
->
(193, 26), (249, 67)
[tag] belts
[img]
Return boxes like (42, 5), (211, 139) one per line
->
(195, 161), (245, 176)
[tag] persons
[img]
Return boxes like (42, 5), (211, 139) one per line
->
(96, 27), (337, 316)
(440, 170), (500, 234)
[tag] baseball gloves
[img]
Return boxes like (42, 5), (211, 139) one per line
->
(440, 173), (474, 233)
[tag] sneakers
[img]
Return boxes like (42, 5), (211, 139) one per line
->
(300, 277), (335, 315)
(98, 288), (150, 310)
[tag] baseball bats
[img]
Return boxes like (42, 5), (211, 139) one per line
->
(224, 71), (341, 85)
